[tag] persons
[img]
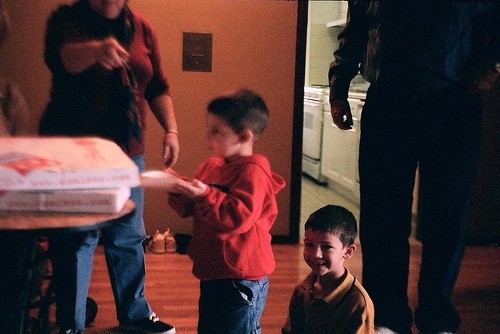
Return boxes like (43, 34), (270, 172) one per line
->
(164, 88), (286, 334)
(329, 0), (500, 334)
(39, 0), (179, 334)
(282, 205), (375, 334)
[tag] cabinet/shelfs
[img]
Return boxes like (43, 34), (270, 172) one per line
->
(321, 90), (366, 207)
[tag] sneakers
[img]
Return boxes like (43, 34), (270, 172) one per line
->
(118, 312), (175, 334)
(59, 328), (83, 334)
(165, 228), (176, 252)
(149, 230), (165, 253)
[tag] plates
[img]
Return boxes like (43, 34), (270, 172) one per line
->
(140, 171), (192, 193)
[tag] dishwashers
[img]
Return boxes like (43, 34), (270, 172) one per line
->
(302, 87), (330, 181)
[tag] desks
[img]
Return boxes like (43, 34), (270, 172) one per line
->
(0, 198), (137, 334)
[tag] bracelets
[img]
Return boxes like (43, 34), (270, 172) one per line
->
(164, 130), (180, 137)
(489, 60), (500, 73)
